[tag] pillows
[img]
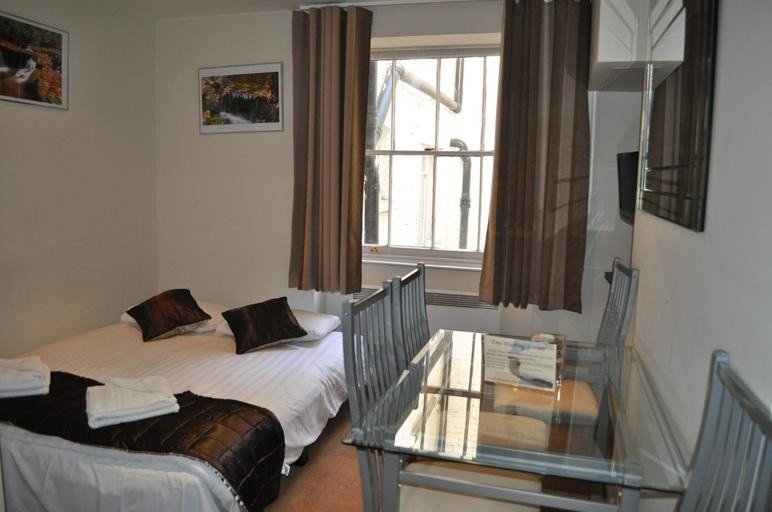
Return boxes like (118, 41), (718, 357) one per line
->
(119, 287), (340, 355)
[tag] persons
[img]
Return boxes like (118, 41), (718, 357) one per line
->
(203, 80), (273, 120)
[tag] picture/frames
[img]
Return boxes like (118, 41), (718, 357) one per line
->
(197, 61), (286, 133)
(1, 9), (69, 111)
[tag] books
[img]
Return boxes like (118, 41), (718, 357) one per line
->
(484, 334), (558, 393)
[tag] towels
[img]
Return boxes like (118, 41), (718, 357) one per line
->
(1, 355), (53, 398)
(84, 373), (180, 431)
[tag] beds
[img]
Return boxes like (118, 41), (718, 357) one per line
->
(1, 322), (348, 509)
